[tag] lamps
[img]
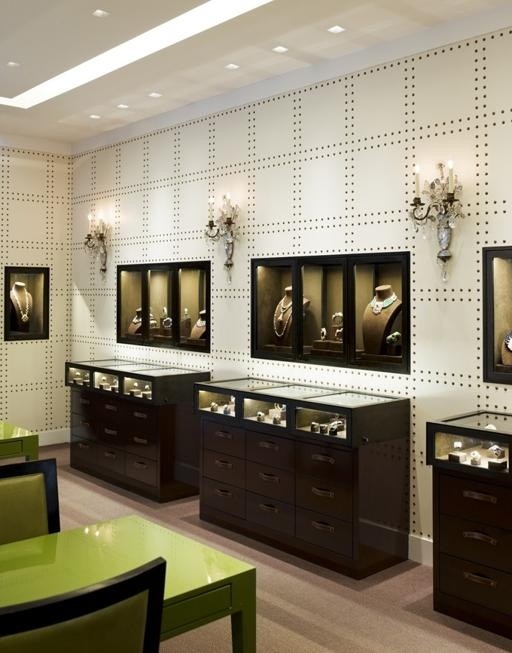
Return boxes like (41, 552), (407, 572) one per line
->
(205, 194), (239, 286)
(84, 214), (109, 282)
(409, 160), (465, 283)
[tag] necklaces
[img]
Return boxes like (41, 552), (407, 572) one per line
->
(12, 287), (32, 322)
(273, 293), (297, 337)
(369, 292), (397, 314)
(130, 314), (206, 332)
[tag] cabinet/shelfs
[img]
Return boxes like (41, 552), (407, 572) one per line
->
(251, 251), (410, 374)
(426, 432), (512, 642)
(4, 266), (49, 342)
(482, 245), (512, 386)
(116, 260), (210, 354)
